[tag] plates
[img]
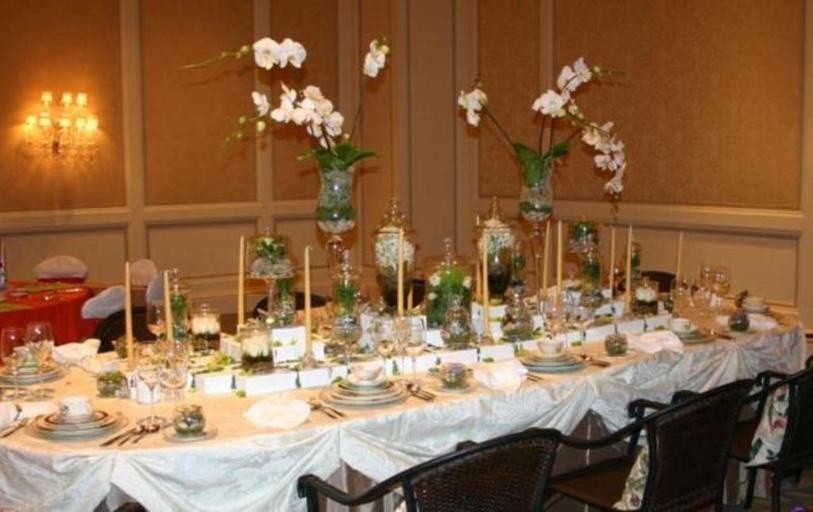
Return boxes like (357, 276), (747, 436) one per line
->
(0, 362), (62, 384)
(740, 303), (768, 313)
(27, 409), (130, 443)
(317, 376), (409, 410)
(166, 425), (217, 443)
(600, 349), (637, 359)
(677, 328), (718, 344)
(428, 378), (478, 394)
(522, 353), (586, 371)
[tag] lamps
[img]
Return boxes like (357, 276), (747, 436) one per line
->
(17, 90), (103, 164)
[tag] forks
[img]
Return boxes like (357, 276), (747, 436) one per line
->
(706, 326), (734, 341)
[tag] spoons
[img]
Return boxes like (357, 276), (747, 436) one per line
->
(307, 396), (344, 421)
(97, 421), (160, 449)
(407, 382), (437, 402)
(580, 354), (610, 368)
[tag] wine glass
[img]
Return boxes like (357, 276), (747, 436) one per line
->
(670, 261), (731, 309)
(131, 298), (194, 422)
(330, 293), (425, 383)
(539, 290), (596, 345)
(1, 319), (56, 402)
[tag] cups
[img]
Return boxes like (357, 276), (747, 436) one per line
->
(536, 339), (564, 357)
(634, 277), (660, 316)
(55, 395), (90, 421)
(236, 323), (272, 374)
(728, 310), (748, 332)
(667, 316), (691, 333)
(743, 294), (764, 306)
(189, 303), (221, 352)
(171, 405), (207, 437)
(602, 332), (628, 354)
(439, 362), (473, 388)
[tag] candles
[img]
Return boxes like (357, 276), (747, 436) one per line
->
(122, 214), (685, 366)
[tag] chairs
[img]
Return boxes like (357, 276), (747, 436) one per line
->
(296, 355), (813, 512)
(33, 254), (88, 284)
(95, 307), (166, 353)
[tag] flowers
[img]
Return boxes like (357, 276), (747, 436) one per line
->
(457, 54), (628, 199)
(178, 32), (391, 170)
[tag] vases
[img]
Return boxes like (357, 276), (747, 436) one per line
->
(517, 165), (555, 223)
(313, 171), (359, 240)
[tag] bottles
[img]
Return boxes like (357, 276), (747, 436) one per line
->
(499, 289), (535, 342)
(438, 292), (472, 349)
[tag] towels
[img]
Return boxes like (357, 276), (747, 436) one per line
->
(626, 329), (685, 357)
(472, 357), (529, 396)
(243, 393), (312, 431)
(744, 312), (779, 332)
(51, 337), (102, 365)
